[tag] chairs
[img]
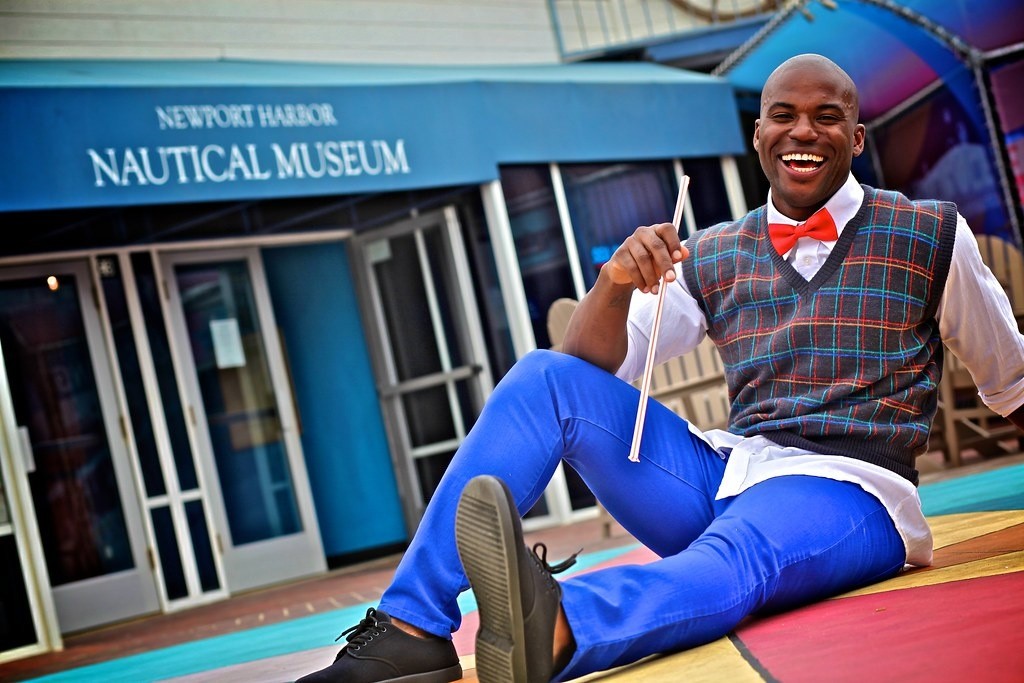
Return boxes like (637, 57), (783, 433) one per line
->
(548, 299), (733, 540)
(932, 234), (1024, 468)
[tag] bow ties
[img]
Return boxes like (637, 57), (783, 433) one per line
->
(769, 207), (839, 257)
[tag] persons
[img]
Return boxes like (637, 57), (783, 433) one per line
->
(298, 53), (1024, 683)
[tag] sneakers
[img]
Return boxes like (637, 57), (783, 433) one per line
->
(294, 607), (463, 683)
(455, 474), (584, 682)
